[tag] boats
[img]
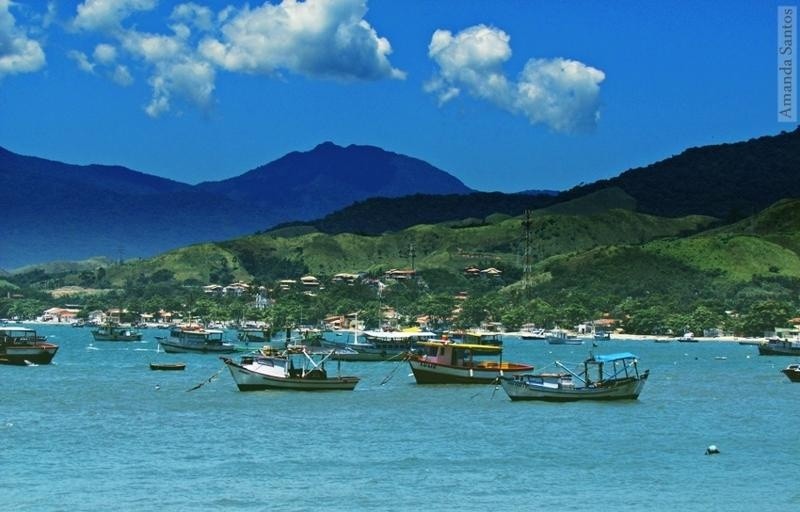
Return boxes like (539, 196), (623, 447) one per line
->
(544, 331), (584, 345)
(222, 327), (315, 355)
(329, 329), (437, 361)
(156, 324), (239, 354)
(220, 343), (359, 391)
(758, 334), (800, 356)
(595, 331), (611, 342)
(91, 326), (144, 342)
(655, 339), (670, 344)
(521, 327), (550, 339)
(1, 319), (8, 326)
(417, 329), (504, 355)
(781, 364), (800, 383)
(500, 352), (650, 402)
(739, 340), (761, 346)
(678, 338), (698, 342)
(715, 356), (728, 360)
(0, 327), (59, 364)
(72, 319), (170, 329)
(300, 328), (325, 341)
(407, 339), (534, 383)
(151, 362), (185, 371)
